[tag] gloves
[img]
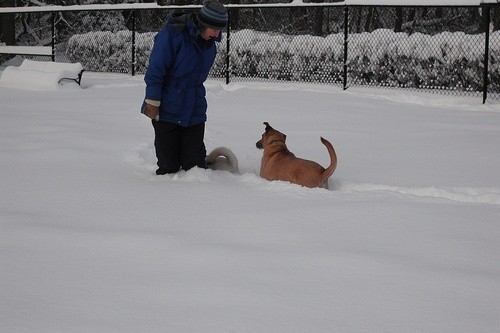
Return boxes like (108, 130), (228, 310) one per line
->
(143, 99), (161, 121)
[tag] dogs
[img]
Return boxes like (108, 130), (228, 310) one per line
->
(204, 146), (240, 174)
(256, 122), (337, 191)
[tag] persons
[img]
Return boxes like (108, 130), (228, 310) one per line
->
(140, 0), (229, 176)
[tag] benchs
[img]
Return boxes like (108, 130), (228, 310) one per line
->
(57, 69), (86, 86)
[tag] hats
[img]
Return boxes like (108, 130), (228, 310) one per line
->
(197, 0), (228, 29)
